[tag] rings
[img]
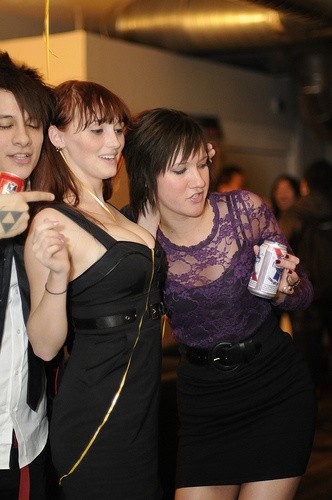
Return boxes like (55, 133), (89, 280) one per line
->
(286, 275), (301, 286)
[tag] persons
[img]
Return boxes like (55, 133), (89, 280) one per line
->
(124, 108), (316, 500)
(0, 52), (55, 500)
(24, 81), (215, 500)
(212, 156), (332, 396)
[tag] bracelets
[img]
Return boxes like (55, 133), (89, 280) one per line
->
(45, 282), (67, 295)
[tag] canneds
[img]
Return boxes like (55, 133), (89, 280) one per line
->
(0, 171), (26, 194)
(248, 239), (287, 298)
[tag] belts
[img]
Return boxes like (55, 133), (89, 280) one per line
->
(181, 324), (274, 374)
(74, 302), (164, 329)
